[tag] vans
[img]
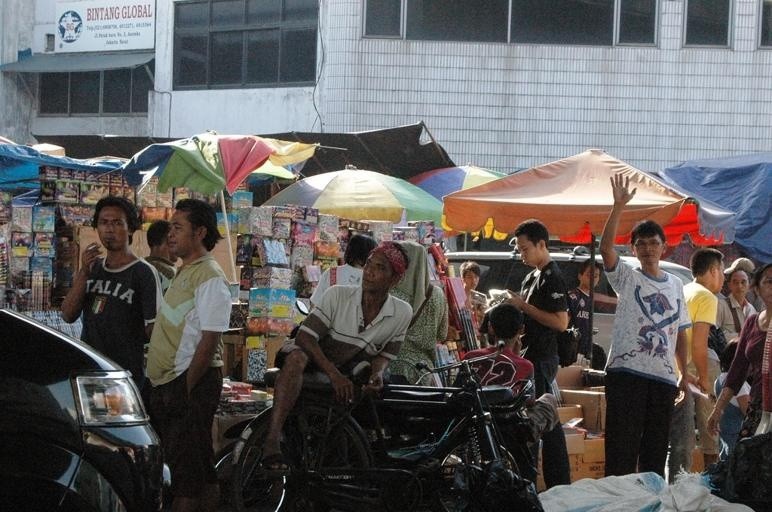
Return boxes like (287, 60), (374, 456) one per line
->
(431, 243), (699, 374)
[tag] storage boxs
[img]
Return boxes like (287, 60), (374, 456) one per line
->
(529, 364), (706, 494)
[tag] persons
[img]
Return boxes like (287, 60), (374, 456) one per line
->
(63, 197), (618, 511)
(714, 268), (757, 345)
(707, 262), (772, 436)
(711, 339), (751, 461)
(721, 257), (763, 312)
(599, 172), (697, 481)
(675, 247), (726, 477)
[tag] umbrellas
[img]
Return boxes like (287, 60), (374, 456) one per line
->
(116, 129), (318, 285)
(443, 147), (737, 369)
(409, 161), (509, 203)
(257, 164), (444, 228)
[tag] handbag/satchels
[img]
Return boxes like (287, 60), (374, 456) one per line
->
(708, 431), (772, 512)
(558, 328), (581, 368)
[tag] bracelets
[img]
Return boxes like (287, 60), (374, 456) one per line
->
(713, 404), (722, 412)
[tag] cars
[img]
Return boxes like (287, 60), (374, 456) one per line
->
(0, 307), (179, 512)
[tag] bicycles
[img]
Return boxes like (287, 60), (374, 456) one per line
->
(228, 332), (546, 512)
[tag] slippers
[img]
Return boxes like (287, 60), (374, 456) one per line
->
(261, 454), (289, 470)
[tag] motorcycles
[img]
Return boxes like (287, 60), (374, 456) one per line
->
(264, 295), (542, 509)
